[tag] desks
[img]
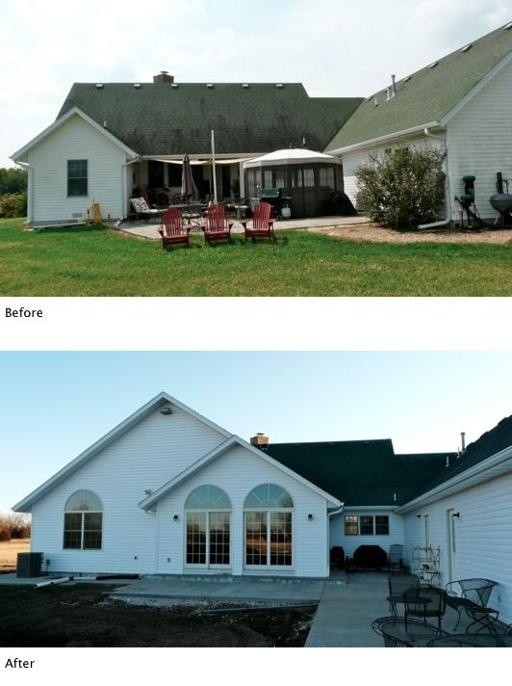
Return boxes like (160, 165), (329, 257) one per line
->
(369, 615), (448, 647)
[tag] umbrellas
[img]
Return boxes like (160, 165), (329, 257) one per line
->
(178, 152), (199, 224)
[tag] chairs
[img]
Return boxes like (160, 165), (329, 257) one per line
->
(130, 189), (170, 221)
(158, 202), (274, 248)
(386, 544), (405, 570)
(385, 571), (499, 647)
